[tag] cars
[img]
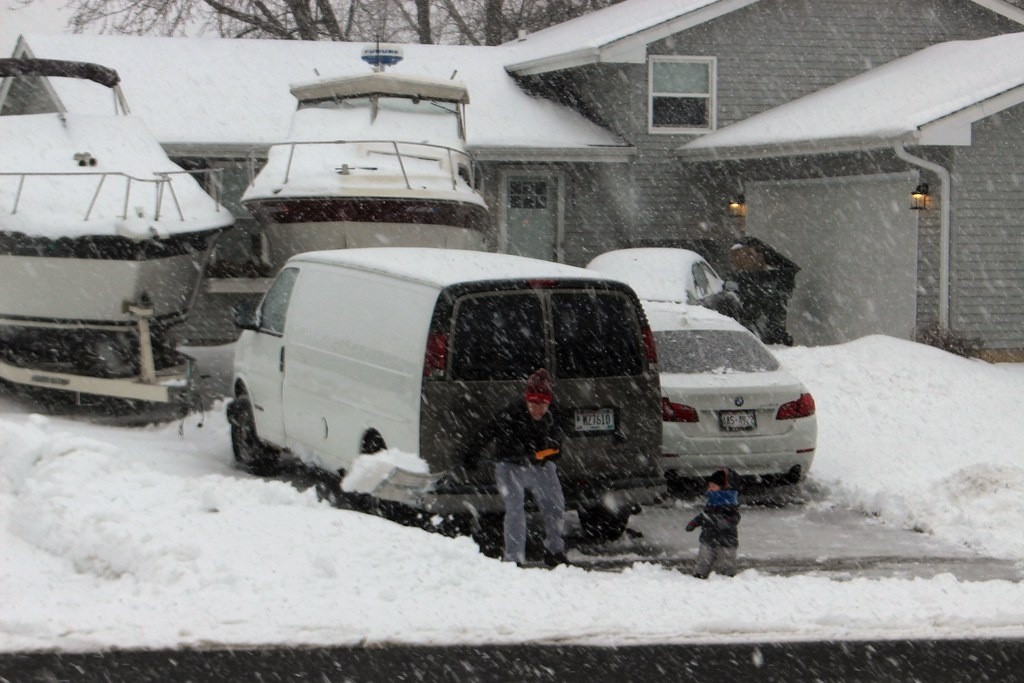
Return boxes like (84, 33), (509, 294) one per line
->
(636, 303), (818, 494)
(581, 251), (747, 330)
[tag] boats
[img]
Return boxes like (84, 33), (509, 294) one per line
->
(240, 35), (493, 271)
(0, 58), (232, 328)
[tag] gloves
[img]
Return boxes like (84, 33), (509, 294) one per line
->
(461, 452), (479, 471)
(686, 521), (696, 531)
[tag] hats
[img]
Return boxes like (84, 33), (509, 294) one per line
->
(709, 469), (728, 486)
(526, 368), (552, 404)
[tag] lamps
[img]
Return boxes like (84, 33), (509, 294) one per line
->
(728, 193), (743, 217)
(910, 183), (927, 209)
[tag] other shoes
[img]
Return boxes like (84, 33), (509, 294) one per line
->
(694, 574), (706, 579)
(544, 551), (576, 567)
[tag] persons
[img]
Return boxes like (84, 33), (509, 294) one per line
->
(686, 469), (741, 580)
(463, 368), (573, 567)
(717, 235), (802, 346)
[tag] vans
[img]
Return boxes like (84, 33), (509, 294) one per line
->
(226, 247), (666, 545)
